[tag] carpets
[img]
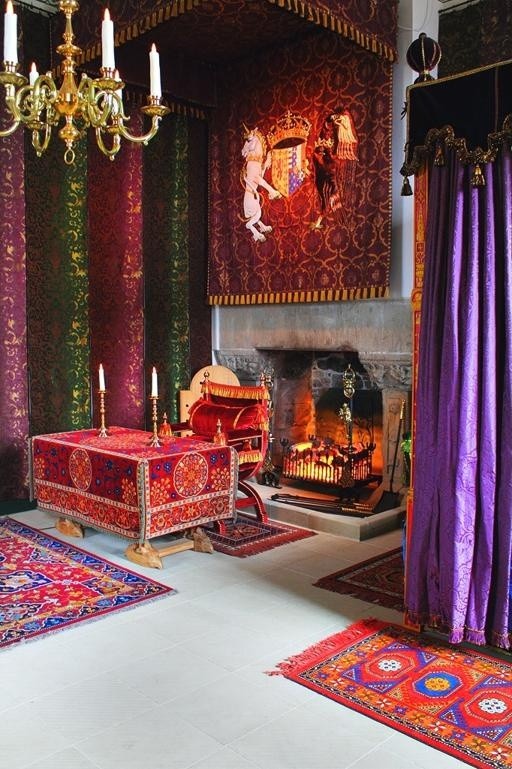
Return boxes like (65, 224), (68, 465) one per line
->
(1, 517), (178, 649)
(171, 512), (319, 559)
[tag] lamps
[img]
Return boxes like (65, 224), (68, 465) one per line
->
(1, 0), (171, 163)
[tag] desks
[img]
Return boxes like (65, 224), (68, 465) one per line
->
(31, 426), (235, 569)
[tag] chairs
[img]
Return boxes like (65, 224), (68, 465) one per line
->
(160, 367), (269, 533)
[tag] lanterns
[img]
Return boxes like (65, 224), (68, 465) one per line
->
(404, 30), (444, 77)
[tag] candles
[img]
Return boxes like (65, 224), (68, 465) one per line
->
(151, 367), (158, 397)
(98, 364), (106, 390)
